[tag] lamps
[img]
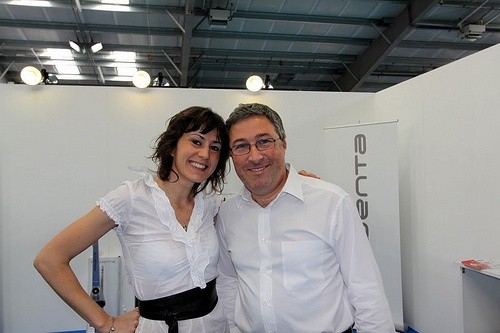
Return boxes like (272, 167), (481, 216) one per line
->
(206, 6), (236, 30)
(459, 18), (487, 43)
(67, 39), (103, 54)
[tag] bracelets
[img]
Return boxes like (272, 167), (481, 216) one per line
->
(106, 317), (115, 333)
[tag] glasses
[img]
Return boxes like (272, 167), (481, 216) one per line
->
(229, 138), (280, 156)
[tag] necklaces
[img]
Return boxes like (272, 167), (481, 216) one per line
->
(165, 181), (190, 232)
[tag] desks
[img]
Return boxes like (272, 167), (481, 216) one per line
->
(455, 261), (500, 333)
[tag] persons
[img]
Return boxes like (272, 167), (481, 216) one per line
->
(214, 103), (396, 333)
(33, 106), (320, 333)
